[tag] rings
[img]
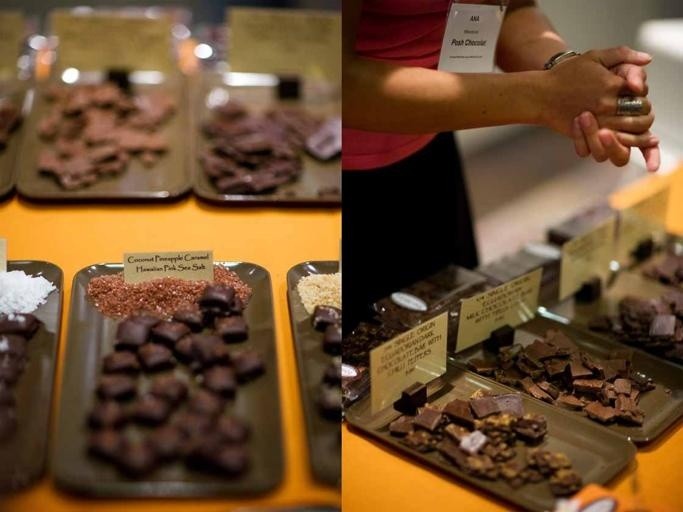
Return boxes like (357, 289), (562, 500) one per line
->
(616, 97), (644, 116)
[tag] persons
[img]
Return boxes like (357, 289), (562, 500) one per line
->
(342, 0), (661, 339)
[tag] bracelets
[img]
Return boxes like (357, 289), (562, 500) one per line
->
(543, 50), (581, 70)
